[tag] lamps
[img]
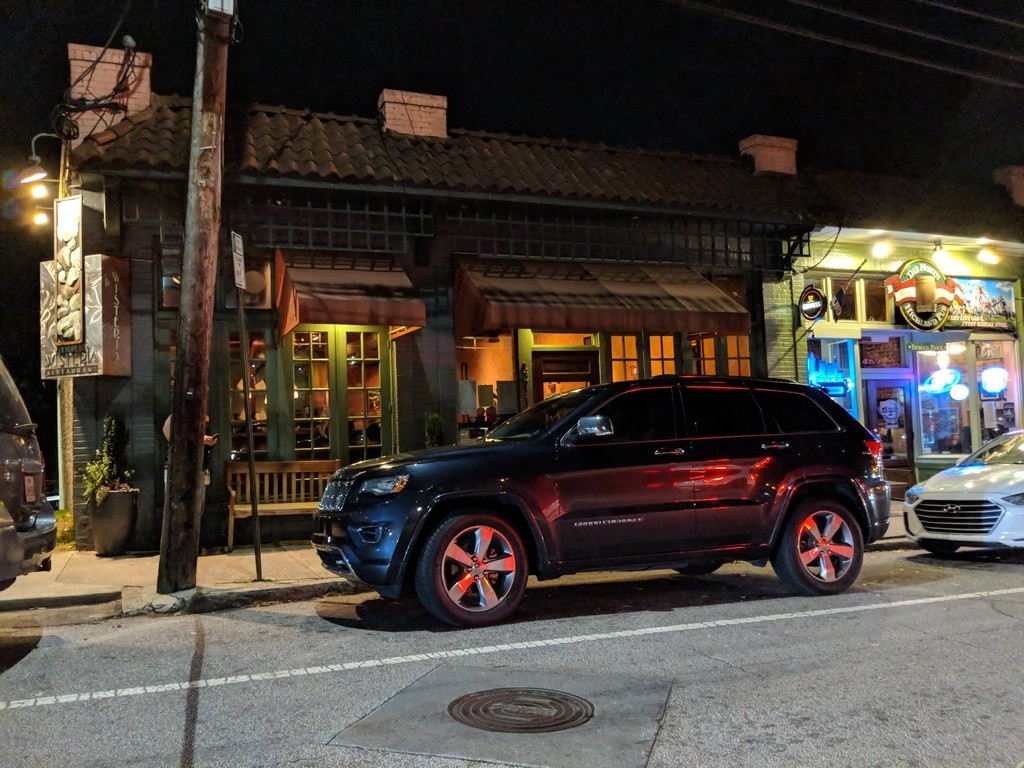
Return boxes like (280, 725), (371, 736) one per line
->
(20, 132), (63, 186)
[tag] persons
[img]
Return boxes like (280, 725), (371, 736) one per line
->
(163, 413), (218, 519)
(232, 406), (499, 466)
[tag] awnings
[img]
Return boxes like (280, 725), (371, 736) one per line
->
(449, 252), (751, 339)
(276, 248), (426, 343)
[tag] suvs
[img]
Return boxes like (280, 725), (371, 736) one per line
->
(309, 371), (892, 631)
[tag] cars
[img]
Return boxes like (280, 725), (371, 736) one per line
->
(0, 358), (57, 592)
(902, 428), (1024, 566)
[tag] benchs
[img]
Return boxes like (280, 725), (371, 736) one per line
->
(223, 458), (341, 553)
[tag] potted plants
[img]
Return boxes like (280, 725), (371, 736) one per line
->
(82, 411), (140, 558)
